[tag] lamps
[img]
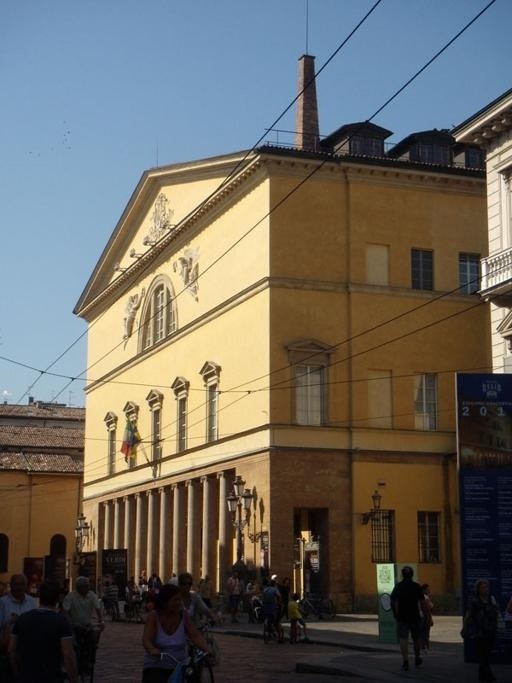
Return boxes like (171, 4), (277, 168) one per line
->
(362, 489), (382, 525)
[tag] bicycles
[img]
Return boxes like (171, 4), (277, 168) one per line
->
(158, 650), (208, 682)
(299, 590), (337, 620)
(74, 623), (101, 682)
(101, 597), (117, 621)
(196, 620), (216, 682)
(262, 620), (285, 644)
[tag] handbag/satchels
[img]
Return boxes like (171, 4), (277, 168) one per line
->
(193, 620), (222, 667)
(490, 594), (506, 635)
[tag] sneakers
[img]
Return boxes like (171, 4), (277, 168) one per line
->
(400, 647), (434, 672)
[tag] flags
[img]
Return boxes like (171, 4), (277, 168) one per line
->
(120, 418), (142, 469)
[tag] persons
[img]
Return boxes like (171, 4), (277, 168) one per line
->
(506, 597), (512, 614)
(460, 578), (504, 683)
(390, 567), (434, 673)
(420, 583), (433, 650)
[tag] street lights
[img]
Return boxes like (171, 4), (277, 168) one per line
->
(72, 512), (91, 552)
(226, 472), (255, 562)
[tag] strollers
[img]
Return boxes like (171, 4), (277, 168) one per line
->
(248, 593), (265, 624)
